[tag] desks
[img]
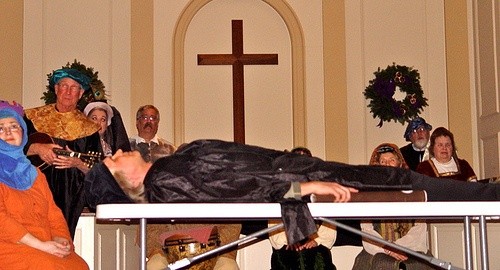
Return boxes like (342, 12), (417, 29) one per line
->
(96, 201), (500, 270)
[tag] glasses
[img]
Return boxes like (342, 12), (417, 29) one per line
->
(139, 116), (157, 121)
(0, 124), (21, 134)
(412, 127), (427, 133)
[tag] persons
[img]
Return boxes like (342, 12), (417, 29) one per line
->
(133, 143), (242, 270)
(23, 62), (175, 239)
(266, 147), (337, 270)
(352, 143), (445, 270)
(414, 127), (479, 202)
(70, 139), (500, 239)
(0, 100), (89, 270)
(400, 118), (437, 171)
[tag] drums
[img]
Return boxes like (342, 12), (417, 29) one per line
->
(163, 226), (221, 270)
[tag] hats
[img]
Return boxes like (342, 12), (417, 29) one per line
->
(404, 117), (432, 141)
(83, 102), (114, 126)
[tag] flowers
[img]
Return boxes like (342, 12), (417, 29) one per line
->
(362, 62), (430, 128)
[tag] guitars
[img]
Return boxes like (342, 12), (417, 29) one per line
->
(23, 133), (102, 172)
